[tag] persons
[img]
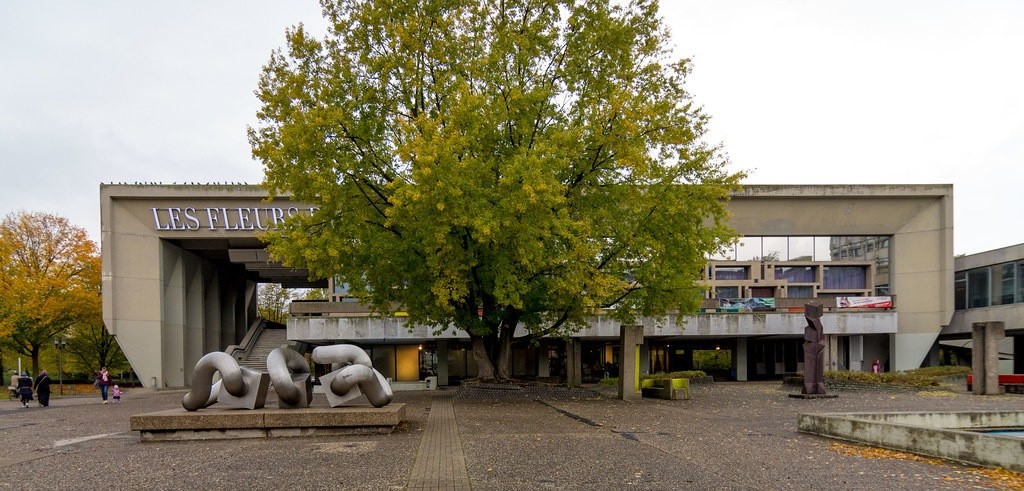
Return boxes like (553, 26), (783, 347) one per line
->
(871, 358), (880, 373)
(110, 385), (123, 403)
(17, 370), (33, 408)
(33, 369), (52, 407)
(10, 370), (20, 399)
(96, 365), (114, 404)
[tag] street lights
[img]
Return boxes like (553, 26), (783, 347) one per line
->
(54, 335), (66, 395)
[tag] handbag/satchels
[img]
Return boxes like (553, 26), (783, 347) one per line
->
(36, 376), (47, 392)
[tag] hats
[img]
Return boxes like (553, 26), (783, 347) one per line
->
(21, 372), (29, 376)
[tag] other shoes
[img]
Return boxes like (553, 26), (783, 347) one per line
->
(22, 402), (28, 408)
(103, 400), (108, 405)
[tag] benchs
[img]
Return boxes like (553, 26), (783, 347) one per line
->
(642, 378), (665, 399)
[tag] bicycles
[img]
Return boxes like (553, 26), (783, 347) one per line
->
(8, 386), (22, 401)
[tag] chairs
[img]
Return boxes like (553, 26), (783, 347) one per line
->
(665, 378), (689, 400)
